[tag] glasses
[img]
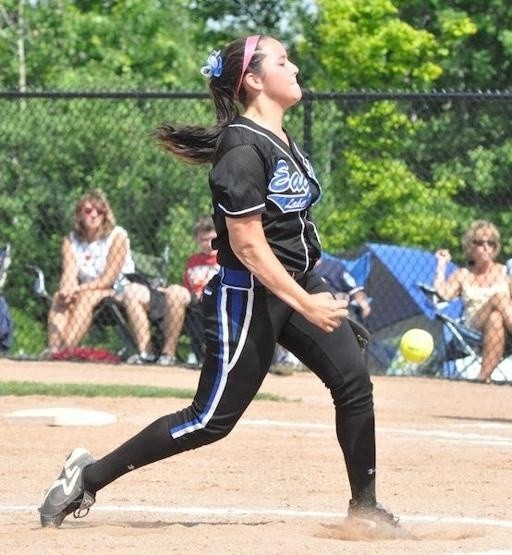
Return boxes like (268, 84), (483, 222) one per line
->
(471, 237), (500, 248)
(80, 206), (108, 217)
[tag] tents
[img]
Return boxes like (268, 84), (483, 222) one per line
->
(344, 239), (466, 373)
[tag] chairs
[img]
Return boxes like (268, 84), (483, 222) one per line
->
(421, 255), (512, 386)
(108, 267), (207, 372)
(23, 243), (168, 351)
(0, 241), (13, 356)
(320, 247), (374, 326)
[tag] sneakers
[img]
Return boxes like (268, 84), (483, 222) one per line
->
(122, 353), (158, 367)
(154, 351), (177, 368)
(37, 445), (98, 531)
(346, 500), (419, 541)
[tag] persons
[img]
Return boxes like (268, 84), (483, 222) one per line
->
(432, 218), (512, 383)
(274, 252), (372, 370)
(36, 186), (135, 360)
(28, 33), (420, 544)
(155, 213), (218, 368)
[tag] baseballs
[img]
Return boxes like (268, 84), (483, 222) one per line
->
(402, 328), (433, 363)
